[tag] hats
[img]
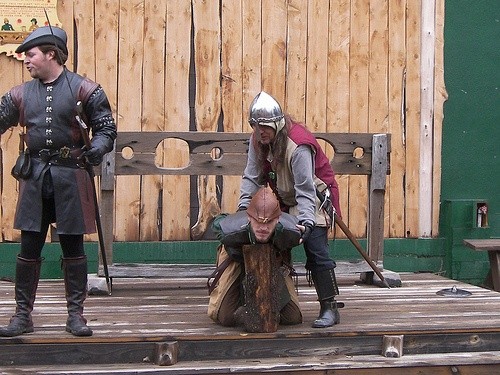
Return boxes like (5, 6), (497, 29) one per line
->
(14, 26), (69, 56)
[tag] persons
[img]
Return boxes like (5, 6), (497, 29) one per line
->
(208, 184), (302, 326)
(238, 92), (345, 328)
(0, 24), (118, 336)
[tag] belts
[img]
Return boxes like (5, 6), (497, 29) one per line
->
(29, 149), (83, 168)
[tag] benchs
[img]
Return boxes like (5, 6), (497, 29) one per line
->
(464, 239), (500, 292)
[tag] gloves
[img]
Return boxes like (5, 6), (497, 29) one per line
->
(84, 134), (113, 166)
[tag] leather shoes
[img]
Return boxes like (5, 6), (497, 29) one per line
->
(313, 298), (345, 328)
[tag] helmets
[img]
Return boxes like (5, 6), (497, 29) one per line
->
(247, 91), (284, 122)
(245, 181), (283, 224)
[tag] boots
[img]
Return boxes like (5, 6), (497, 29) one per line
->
(0, 253), (44, 337)
(60, 254), (93, 336)
